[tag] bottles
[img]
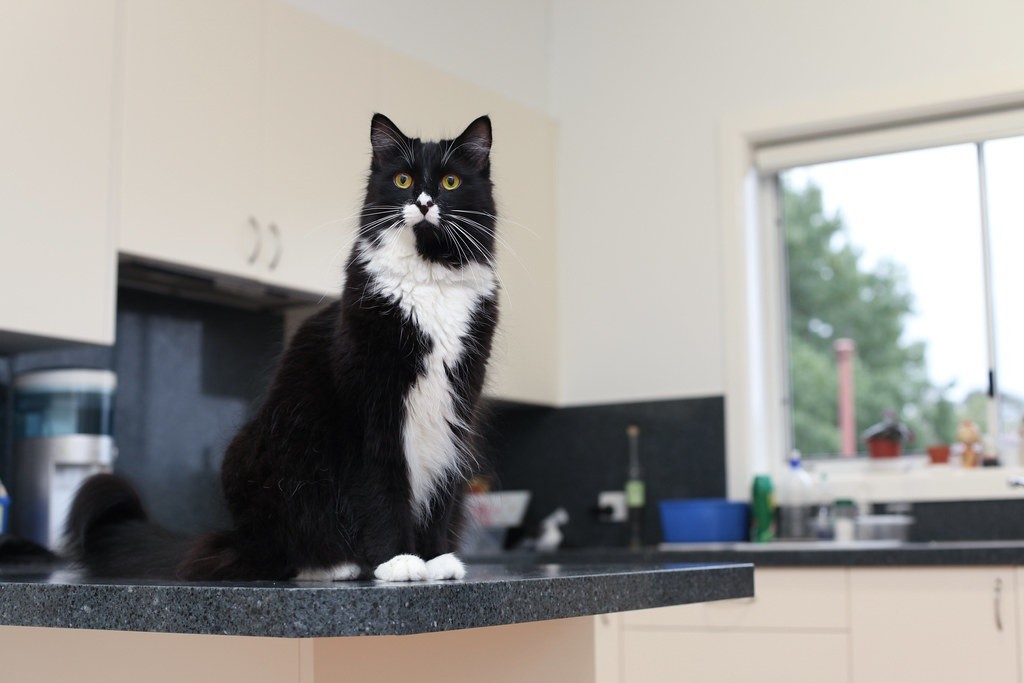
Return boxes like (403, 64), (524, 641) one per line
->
(831, 497), (860, 542)
(783, 450), (807, 539)
(752, 477), (779, 542)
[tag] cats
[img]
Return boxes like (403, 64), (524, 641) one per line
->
(55, 111), (543, 583)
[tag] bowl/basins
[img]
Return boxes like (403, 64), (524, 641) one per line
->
(656, 501), (750, 541)
(856, 515), (918, 543)
(459, 526), (508, 557)
